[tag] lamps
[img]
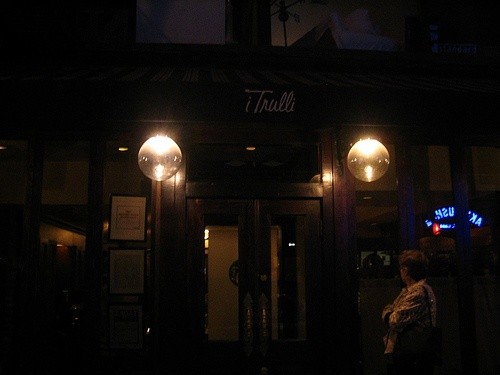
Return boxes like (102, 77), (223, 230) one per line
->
(346, 139), (389, 183)
(138, 133), (182, 181)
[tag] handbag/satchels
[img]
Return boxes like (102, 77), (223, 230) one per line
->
(398, 323), (440, 359)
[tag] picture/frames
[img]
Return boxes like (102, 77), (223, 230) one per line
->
(106, 301), (146, 353)
(108, 247), (147, 295)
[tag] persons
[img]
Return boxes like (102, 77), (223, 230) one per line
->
(380, 250), (439, 375)
